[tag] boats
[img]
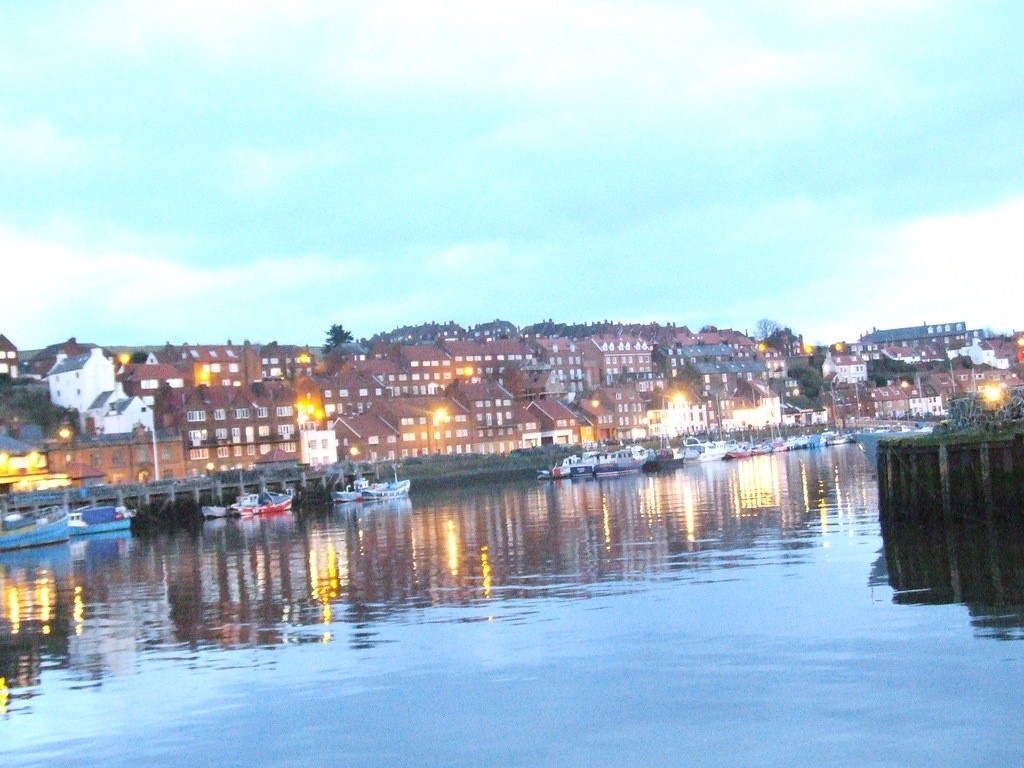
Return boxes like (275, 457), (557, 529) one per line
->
(0, 505), (131, 550)
(331, 472), (411, 503)
(202, 488), (293, 516)
(536, 421), (934, 482)
